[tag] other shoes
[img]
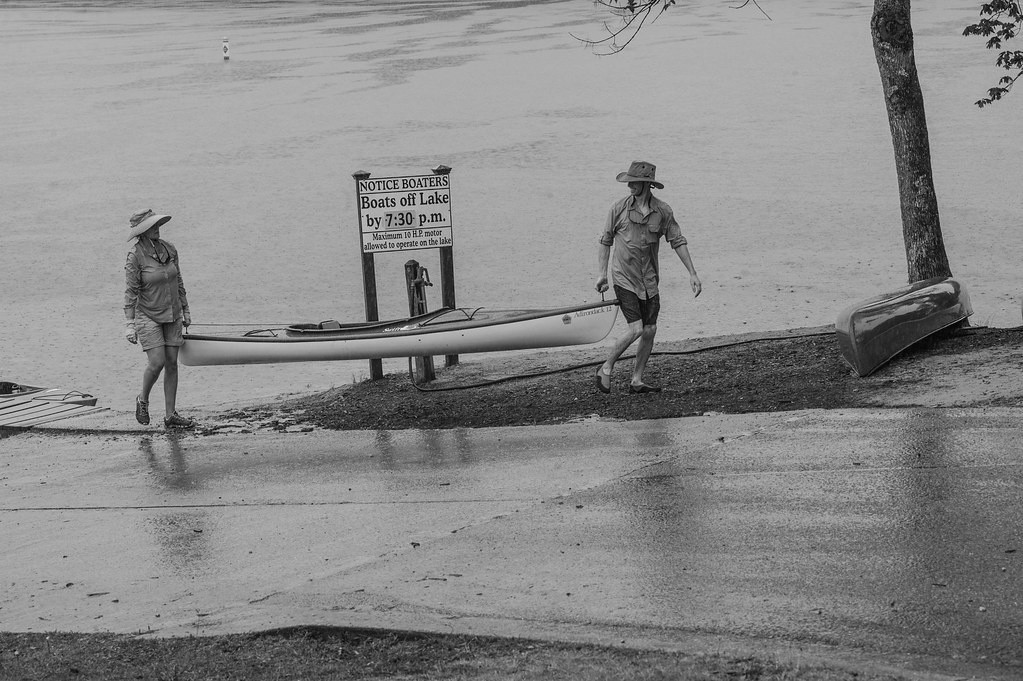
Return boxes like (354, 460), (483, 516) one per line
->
(594, 364), (613, 394)
(629, 381), (661, 393)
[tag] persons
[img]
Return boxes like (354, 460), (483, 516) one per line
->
(595, 159), (702, 394)
(124, 209), (194, 427)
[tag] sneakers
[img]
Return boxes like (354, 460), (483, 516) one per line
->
(163, 411), (193, 428)
(135, 395), (149, 425)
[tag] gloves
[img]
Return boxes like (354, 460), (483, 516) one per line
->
(181, 312), (191, 327)
(125, 320), (137, 345)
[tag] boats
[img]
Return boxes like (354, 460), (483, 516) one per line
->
(178, 299), (619, 366)
(833, 277), (975, 378)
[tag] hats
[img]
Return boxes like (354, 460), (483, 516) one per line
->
(615, 160), (664, 189)
(126, 209), (171, 243)
